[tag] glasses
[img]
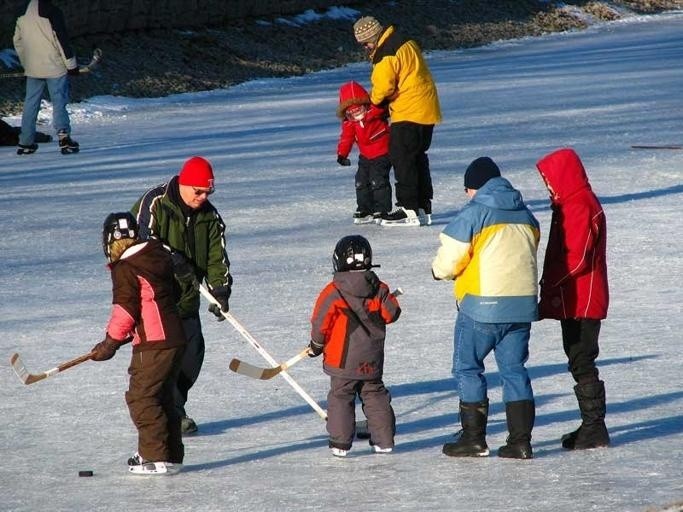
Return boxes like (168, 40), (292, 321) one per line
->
(192, 187), (215, 195)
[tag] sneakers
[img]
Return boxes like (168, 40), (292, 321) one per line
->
(17, 144), (38, 154)
(353, 207), (432, 222)
(128, 452), (183, 473)
(331, 447), (350, 457)
(371, 445), (392, 453)
(59, 135), (79, 150)
(182, 418), (197, 435)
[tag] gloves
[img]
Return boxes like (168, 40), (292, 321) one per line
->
(67, 68), (79, 74)
(308, 340), (324, 358)
(208, 286), (231, 321)
(336, 156), (351, 166)
(91, 332), (122, 361)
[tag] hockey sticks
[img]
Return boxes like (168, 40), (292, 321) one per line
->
(198, 284), (369, 428)
(0, 48), (102, 78)
(230, 287), (404, 380)
(12, 336), (134, 385)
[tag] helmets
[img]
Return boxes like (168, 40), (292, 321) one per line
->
(333, 235), (380, 272)
(103, 212), (139, 257)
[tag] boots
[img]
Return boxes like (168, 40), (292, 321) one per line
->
(562, 381), (610, 449)
(498, 399), (535, 458)
(442, 398), (489, 457)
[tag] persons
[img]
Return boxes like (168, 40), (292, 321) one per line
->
(90, 211), (188, 465)
(354, 16), (441, 222)
(307, 236), (401, 455)
(534, 148), (613, 449)
(336, 81), (391, 219)
(130, 155), (232, 434)
(431, 156), (540, 460)
(7, 0), (79, 152)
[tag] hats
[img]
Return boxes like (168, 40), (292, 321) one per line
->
(179, 156), (214, 188)
(464, 157), (500, 189)
(354, 16), (382, 41)
